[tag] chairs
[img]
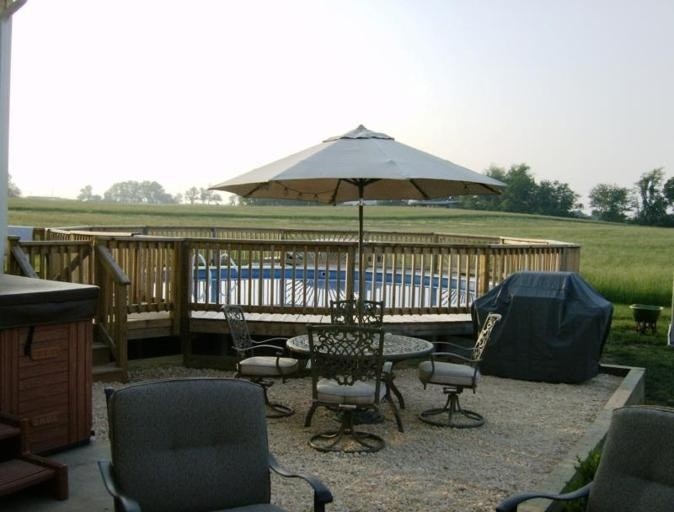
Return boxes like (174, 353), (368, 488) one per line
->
(96, 377), (333, 511)
(493, 405), (674, 512)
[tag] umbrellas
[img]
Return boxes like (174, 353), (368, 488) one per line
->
(205, 124), (508, 328)
